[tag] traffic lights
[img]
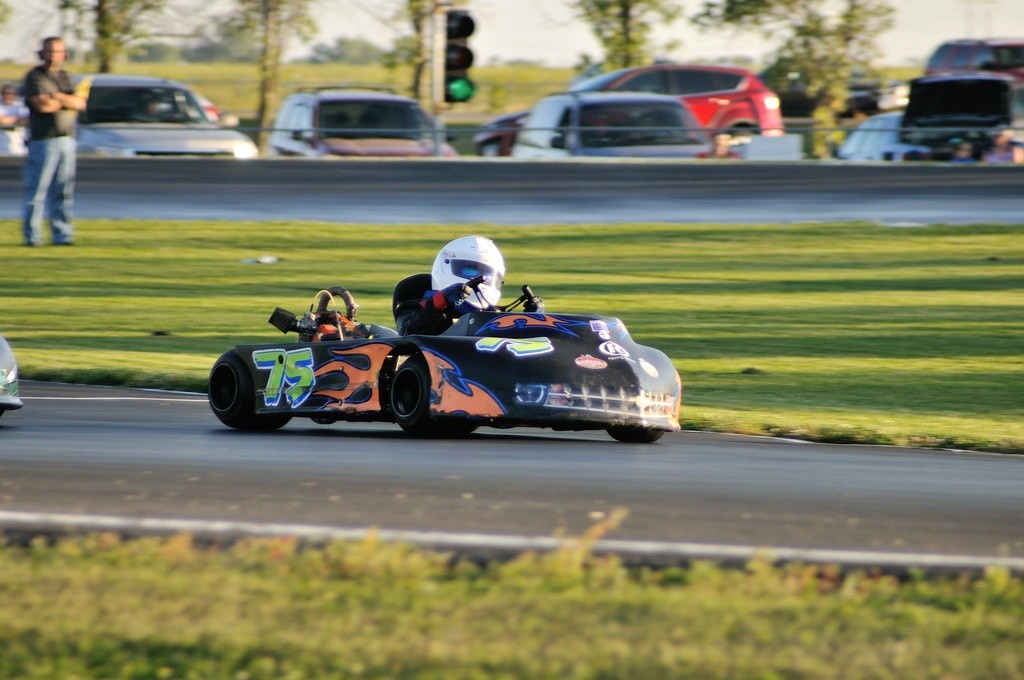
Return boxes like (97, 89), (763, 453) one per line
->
(437, 2), (478, 105)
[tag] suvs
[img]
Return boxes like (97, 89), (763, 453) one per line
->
(265, 85), (462, 163)
(470, 60), (786, 155)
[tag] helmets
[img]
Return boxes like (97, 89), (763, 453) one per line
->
(431, 235), (506, 310)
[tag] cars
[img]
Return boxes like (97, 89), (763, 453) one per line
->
(512, 85), (718, 158)
(833, 33), (1024, 164)
(65, 73), (259, 164)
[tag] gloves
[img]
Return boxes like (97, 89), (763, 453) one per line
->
(442, 283), (473, 306)
(521, 297), (546, 314)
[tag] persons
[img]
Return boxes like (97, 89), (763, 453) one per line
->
(23, 37), (87, 248)
(697, 130), (738, 159)
(394, 235), (505, 336)
(946, 131), (1019, 165)
(0, 83), (33, 159)
